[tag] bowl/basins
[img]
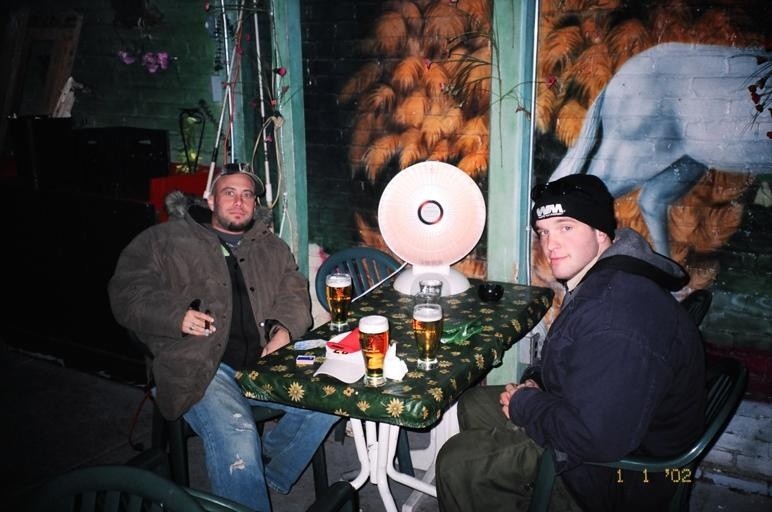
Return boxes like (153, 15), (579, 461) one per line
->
(477, 283), (504, 303)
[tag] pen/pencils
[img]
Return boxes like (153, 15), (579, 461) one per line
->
(205, 308), (211, 337)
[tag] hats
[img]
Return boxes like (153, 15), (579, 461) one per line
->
(210, 163), (265, 195)
(531, 175), (617, 241)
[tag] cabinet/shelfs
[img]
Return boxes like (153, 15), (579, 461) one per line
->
(0, 184), (155, 389)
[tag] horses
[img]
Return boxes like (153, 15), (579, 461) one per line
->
(544, 40), (772, 260)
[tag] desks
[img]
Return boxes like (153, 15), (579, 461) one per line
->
(234, 274), (555, 512)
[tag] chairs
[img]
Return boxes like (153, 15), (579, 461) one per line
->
(520, 290), (712, 391)
(127, 329), (329, 501)
(20, 447), (358, 512)
(530, 357), (748, 512)
(316, 247), (415, 477)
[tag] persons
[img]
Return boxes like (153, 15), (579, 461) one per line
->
(434, 174), (709, 509)
(108, 161), (342, 509)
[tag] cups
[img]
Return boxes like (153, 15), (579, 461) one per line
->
(414, 279), (443, 303)
(326, 273), (353, 331)
(359, 315), (390, 388)
(412, 303), (443, 371)
